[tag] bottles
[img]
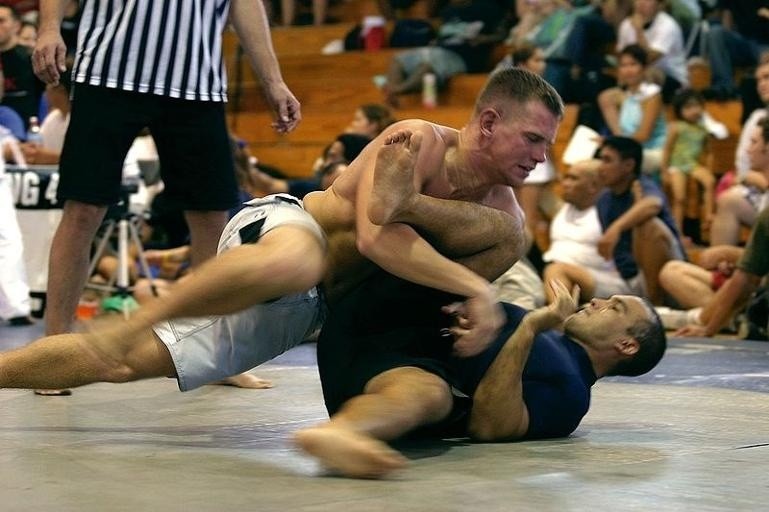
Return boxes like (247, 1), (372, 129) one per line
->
(422, 73), (435, 108)
(746, 188), (764, 212)
(27, 116), (44, 152)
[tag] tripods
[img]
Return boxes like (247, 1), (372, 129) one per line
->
(83, 197), (157, 321)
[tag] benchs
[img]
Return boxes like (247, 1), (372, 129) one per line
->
(218, 1), (744, 244)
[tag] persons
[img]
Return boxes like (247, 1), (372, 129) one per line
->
(0, 66), (565, 391)
(242, 101), (399, 198)
(293, 129), (667, 479)
(1, 1), (192, 304)
(387, 0), (769, 339)
(33, 1), (300, 396)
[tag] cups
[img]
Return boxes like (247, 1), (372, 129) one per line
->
(364, 17), (385, 50)
